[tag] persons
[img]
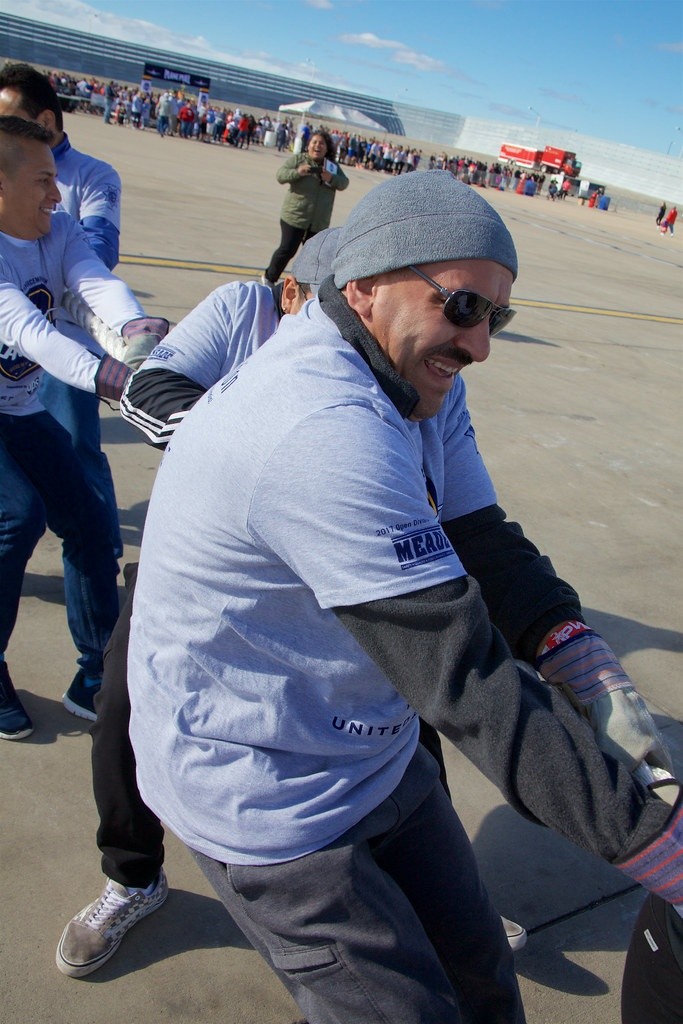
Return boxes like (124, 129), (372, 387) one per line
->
(561, 179), (575, 199)
(429, 151), (546, 195)
(661, 206), (677, 237)
(548, 177), (559, 200)
(656, 202), (667, 228)
(0, 114), (171, 740)
(56, 228), (527, 978)
(262, 130), (350, 287)
(588, 188), (603, 208)
(301, 122), (422, 175)
(277, 117), (293, 151)
(0, 63), (121, 271)
(42, 70), (276, 151)
(127, 169), (683, 1024)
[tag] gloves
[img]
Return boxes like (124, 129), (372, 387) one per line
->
(609, 778), (683, 921)
(121, 316), (169, 370)
(534, 630), (675, 785)
(93, 354), (136, 402)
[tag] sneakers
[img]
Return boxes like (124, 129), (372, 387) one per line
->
(499, 913), (527, 951)
(56, 866), (168, 978)
(62, 672), (102, 721)
(0, 661), (35, 740)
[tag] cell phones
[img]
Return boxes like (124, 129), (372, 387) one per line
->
(307, 167), (323, 174)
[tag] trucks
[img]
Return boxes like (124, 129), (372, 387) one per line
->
(497, 142), (582, 177)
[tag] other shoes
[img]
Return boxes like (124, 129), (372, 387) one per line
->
(262, 275), (275, 289)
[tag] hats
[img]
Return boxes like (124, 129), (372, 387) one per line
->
(290, 226), (341, 298)
(330, 169), (518, 290)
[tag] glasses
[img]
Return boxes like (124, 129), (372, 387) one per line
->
(407, 265), (517, 337)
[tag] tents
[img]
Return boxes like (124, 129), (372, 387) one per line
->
(276, 101), (388, 141)
